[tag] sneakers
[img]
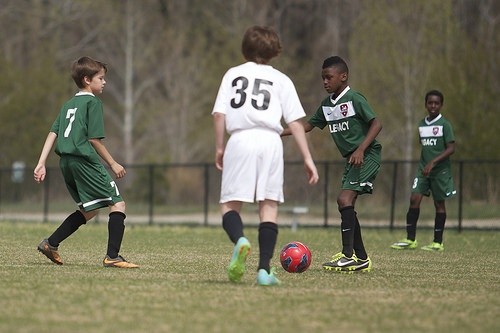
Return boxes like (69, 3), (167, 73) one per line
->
(390, 238), (419, 250)
(354, 256), (372, 272)
(226, 237), (251, 282)
(322, 252), (358, 274)
(421, 240), (444, 252)
(256, 266), (282, 286)
(103, 255), (140, 268)
(38, 239), (64, 265)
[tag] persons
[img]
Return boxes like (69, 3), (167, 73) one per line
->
(211, 26), (318, 289)
(33, 57), (138, 269)
(391, 90), (455, 252)
(279, 56), (382, 273)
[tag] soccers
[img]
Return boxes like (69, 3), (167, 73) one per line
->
(279, 241), (312, 274)
